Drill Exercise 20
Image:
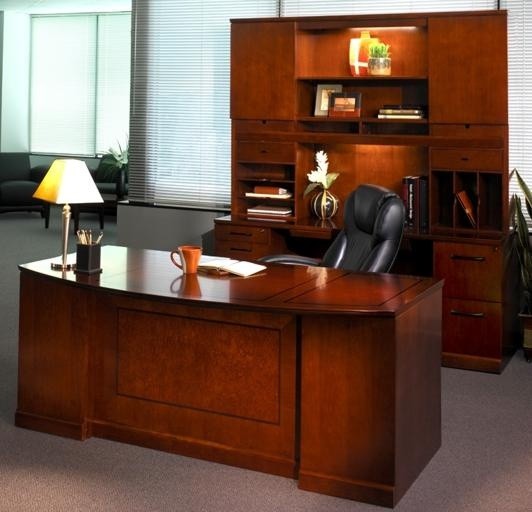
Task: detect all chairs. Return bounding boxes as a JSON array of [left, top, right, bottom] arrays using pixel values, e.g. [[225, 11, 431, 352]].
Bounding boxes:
[[254, 183, 407, 274], [87, 155, 126, 199], [0, 151, 51, 229]]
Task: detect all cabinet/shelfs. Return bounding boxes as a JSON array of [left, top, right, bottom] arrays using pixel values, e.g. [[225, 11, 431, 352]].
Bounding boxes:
[[230, 119, 312, 230], [229, 17, 298, 120], [432, 10, 508, 124], [428, 123, 510, 235], [215, 215, 274, 264], [298, 9, 431, 133], [436, 235, 512, 374]]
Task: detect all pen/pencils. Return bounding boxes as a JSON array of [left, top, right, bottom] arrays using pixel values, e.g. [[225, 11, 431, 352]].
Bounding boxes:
[[74, 228, 105, 245]]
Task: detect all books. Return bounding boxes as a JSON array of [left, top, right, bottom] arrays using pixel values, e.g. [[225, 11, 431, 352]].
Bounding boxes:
[[247, 205, 292, 215], [378, 104, 424, 120], [245, 192, 292, 199], [198, 255, 267, 277], [402, 175, 429, 234]]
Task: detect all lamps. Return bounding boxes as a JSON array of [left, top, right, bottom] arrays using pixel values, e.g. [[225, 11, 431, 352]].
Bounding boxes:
[[31, 155, 105, 270]]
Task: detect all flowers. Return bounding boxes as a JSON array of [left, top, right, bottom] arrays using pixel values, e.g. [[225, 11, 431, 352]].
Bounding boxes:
[[302, 149, 340, 194]]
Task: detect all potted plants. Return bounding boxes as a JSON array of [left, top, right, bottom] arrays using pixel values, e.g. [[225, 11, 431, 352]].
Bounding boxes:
[[509, 166, 532, 362], [367, 41, 391, 75]]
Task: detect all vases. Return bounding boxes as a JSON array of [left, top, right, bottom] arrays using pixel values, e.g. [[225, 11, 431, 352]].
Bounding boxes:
[[312, 189, 338, 220]]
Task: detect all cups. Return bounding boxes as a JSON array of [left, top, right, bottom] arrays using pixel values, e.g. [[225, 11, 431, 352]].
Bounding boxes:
[[171, 246, 202, 275]]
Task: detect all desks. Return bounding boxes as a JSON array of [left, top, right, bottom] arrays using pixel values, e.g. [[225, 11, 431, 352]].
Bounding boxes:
[[71, 193, 118, 235], [14, 243, 447, 510]]
